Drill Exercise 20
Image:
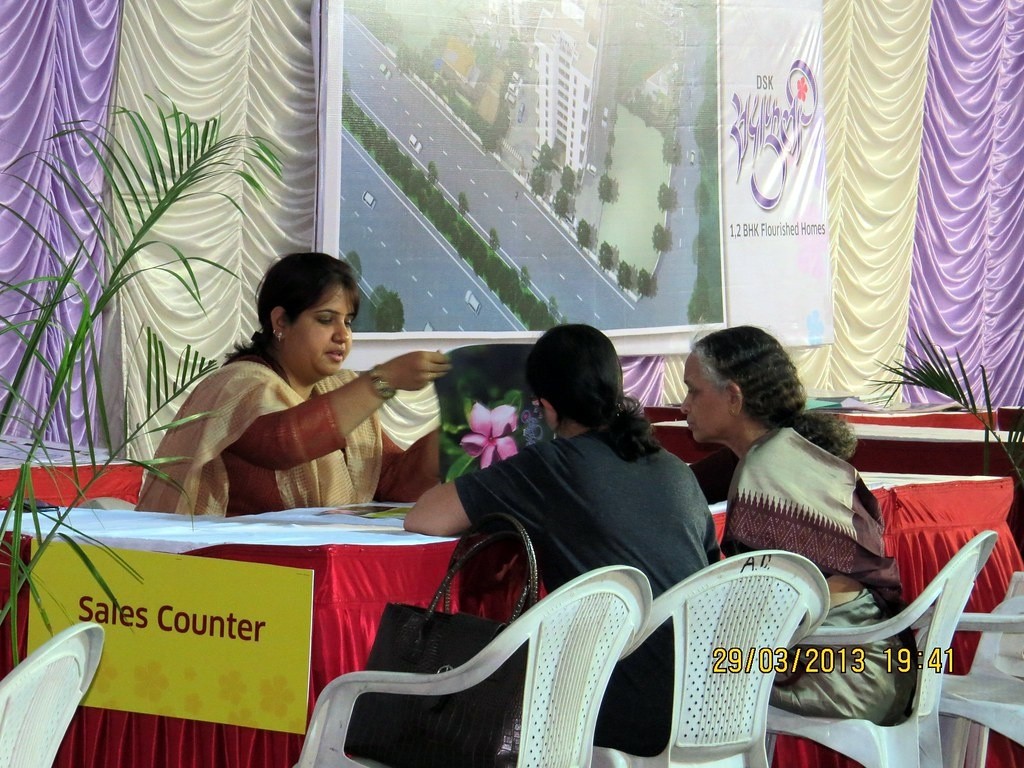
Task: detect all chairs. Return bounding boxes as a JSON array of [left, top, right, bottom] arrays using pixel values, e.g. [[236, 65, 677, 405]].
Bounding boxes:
[[0, 622, 105, 768], [293, 565, 654, 768], [916, 597, 1024, 768], [590, 549, 830, 768], [766, 530, 997, 768]]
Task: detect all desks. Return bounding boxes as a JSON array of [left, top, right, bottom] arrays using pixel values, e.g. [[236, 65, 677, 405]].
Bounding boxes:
[[0, 409, 1024, 764]]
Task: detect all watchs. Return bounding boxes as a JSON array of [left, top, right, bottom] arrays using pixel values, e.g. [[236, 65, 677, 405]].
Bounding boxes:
[[366, 370, 395, 401]]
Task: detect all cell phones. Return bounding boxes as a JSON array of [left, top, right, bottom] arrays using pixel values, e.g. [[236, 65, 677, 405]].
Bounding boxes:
[[23, 498, 59, 512]]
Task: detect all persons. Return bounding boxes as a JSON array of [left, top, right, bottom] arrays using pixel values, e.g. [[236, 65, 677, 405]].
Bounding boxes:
[[679, 325, 917, 725], [134, 252, 451, 537], [404, 324, 721, 752]]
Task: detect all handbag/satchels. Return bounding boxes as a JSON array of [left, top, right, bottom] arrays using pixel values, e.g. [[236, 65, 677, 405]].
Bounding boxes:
[[344, 512, 539, 768]]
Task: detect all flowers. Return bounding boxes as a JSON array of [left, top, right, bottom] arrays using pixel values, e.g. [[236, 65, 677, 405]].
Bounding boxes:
[[460, 402, 520, 470]]
